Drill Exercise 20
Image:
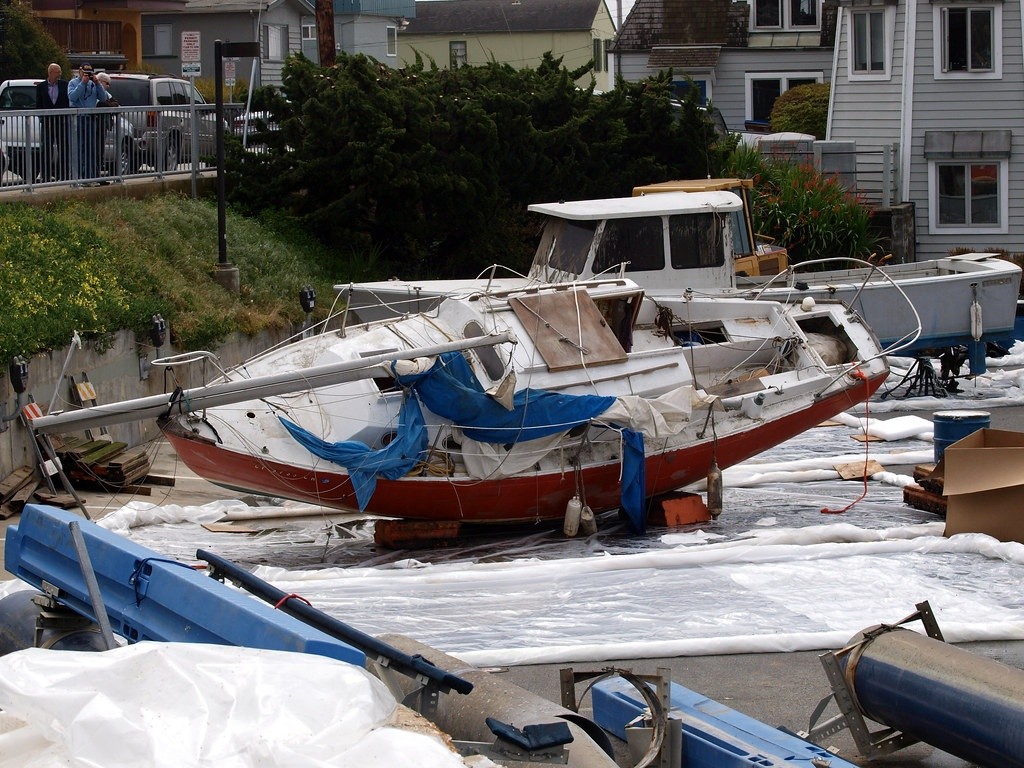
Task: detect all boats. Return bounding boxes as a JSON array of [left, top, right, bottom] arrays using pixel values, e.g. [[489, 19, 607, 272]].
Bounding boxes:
[[334, 191, 1023, 380], [158, 278, 890, 522]]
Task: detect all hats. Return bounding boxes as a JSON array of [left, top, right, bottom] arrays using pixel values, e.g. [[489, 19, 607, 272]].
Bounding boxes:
[[79, 63, 94, 73]]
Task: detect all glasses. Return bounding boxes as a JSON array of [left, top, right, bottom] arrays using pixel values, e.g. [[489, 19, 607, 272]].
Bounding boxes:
[[103, 80, 111, 86]]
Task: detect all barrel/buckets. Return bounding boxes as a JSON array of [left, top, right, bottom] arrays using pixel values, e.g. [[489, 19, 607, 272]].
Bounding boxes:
[[933, 410, 991, 464]]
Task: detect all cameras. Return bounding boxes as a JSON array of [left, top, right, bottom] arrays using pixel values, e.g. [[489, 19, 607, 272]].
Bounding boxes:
[[83, 69, 94, 77]]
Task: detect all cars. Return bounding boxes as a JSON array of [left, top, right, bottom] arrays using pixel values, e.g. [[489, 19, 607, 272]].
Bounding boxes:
[[232, 102, 281, 147]]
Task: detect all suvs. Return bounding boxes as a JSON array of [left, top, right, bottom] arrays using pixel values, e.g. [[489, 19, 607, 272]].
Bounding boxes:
[[95, 71, 230, 172]]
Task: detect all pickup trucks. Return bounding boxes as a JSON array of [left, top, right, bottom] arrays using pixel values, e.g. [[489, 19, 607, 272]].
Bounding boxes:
[[0, 79, 134, 178]]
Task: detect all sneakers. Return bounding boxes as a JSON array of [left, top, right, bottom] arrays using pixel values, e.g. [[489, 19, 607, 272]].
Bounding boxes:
[[80, 182, 100, 187]]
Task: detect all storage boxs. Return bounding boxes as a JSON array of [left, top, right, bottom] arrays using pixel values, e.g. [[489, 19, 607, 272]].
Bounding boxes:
[[944, 428, 1024, 544]]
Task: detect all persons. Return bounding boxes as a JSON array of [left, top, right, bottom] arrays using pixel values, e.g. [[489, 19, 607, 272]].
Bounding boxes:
[[36, 62, 120, 182]]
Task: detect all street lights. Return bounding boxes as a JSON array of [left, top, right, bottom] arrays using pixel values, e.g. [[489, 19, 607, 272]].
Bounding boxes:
[[214, 41, 262, 263]]
